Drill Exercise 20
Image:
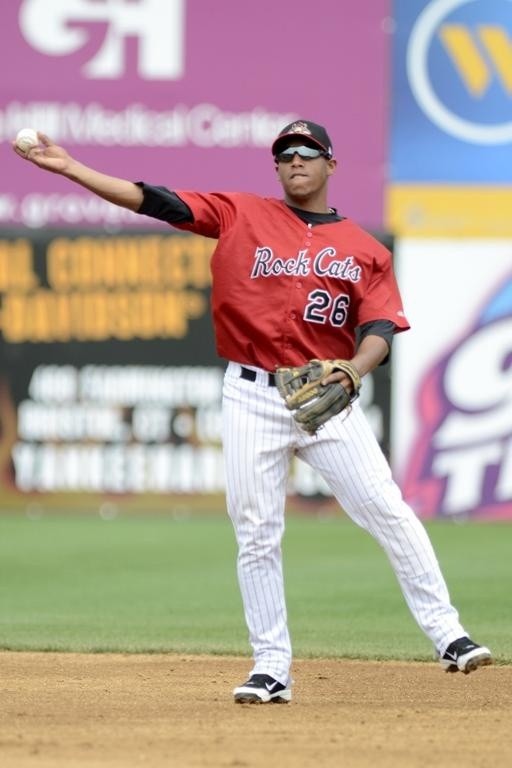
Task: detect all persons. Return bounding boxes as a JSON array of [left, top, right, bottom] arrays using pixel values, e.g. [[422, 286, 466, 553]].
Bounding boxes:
[[12, 120, 494, 705]]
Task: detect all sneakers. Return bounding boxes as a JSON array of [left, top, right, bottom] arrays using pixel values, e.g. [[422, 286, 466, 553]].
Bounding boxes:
[[440, 636, 493, 676], [234, 675, 293, 703]]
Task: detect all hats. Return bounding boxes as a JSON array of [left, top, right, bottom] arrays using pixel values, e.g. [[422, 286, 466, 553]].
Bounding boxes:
[[272, 120, 332, 157]]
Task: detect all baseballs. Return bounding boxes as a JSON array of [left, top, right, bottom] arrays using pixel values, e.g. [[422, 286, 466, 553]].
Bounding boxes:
[[16, 128, 40, 153]]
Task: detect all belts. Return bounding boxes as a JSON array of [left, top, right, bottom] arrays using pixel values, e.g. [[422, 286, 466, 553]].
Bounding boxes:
[[240, 366, 307, 388]]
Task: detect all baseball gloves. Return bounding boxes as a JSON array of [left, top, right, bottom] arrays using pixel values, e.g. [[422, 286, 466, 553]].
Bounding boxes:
[[274, 359, 361, 438]]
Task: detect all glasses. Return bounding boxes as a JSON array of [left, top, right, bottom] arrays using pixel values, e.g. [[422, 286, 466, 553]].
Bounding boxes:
[[274, 143, 330, 164]]
